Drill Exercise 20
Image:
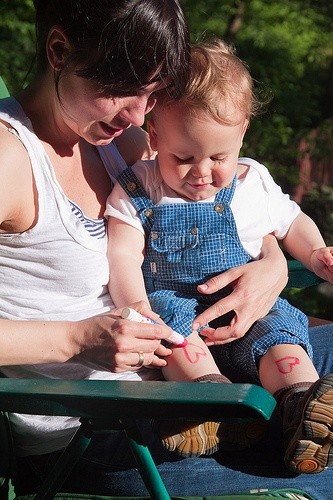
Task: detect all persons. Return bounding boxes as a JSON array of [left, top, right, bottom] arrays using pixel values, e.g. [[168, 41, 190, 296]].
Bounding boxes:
[[104, 37, 333, 473], [0, 0, 332, 500]]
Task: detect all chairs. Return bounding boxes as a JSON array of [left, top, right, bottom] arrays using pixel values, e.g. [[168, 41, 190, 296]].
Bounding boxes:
[[0, 260, 326, 500]]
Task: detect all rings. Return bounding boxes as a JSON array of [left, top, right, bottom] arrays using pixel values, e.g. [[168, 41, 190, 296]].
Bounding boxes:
[[135, 352, 145, 367]]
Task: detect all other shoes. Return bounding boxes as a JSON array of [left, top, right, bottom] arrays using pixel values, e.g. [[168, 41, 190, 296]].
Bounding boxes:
[[160, 373, 263, 458], [279, 372, 333, 475]]
